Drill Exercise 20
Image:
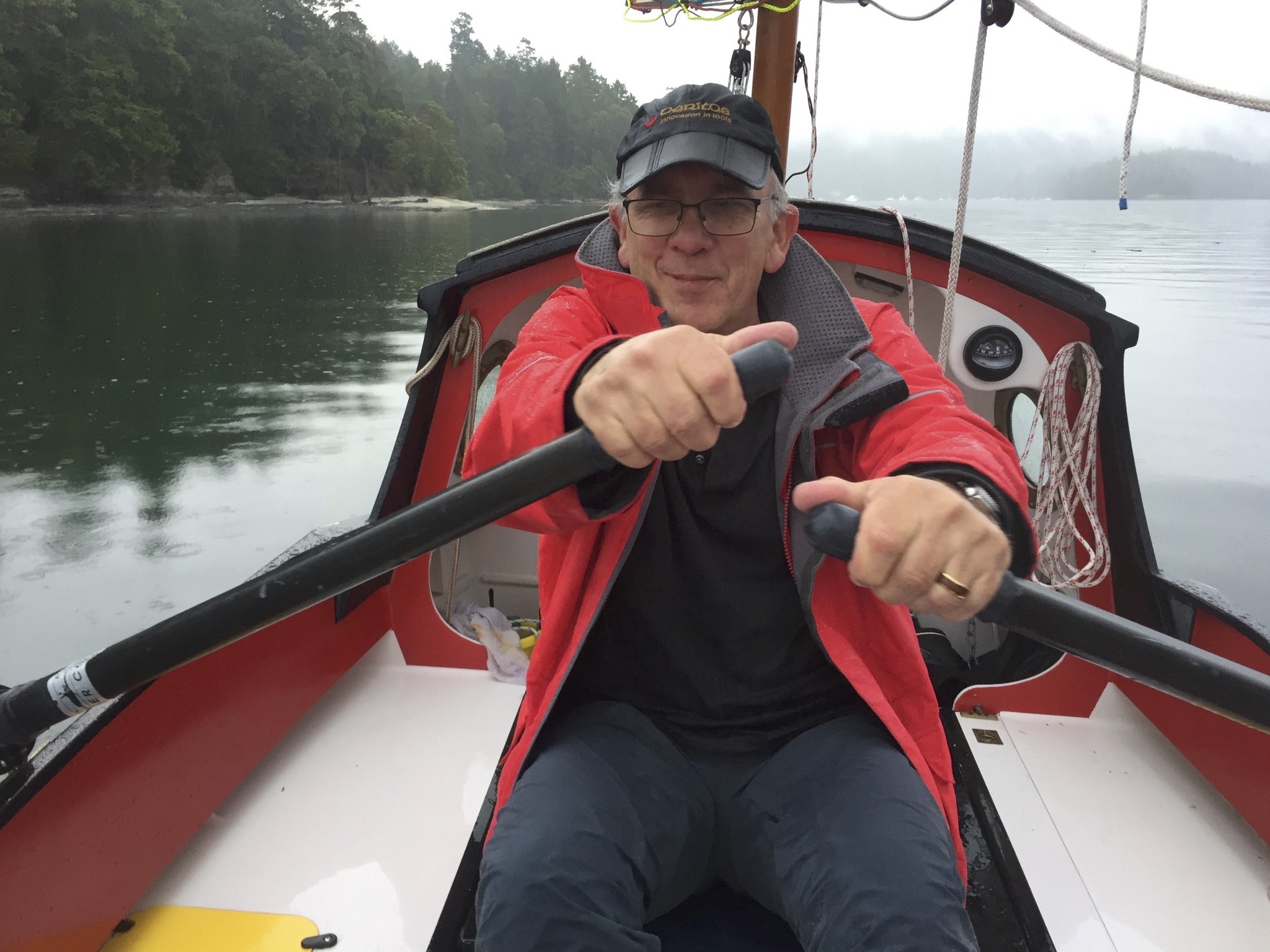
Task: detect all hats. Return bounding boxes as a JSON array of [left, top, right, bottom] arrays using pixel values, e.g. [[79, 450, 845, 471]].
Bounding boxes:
[[616, 83, 785, 191]]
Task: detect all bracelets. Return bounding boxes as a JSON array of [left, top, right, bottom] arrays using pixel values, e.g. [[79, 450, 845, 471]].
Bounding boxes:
[[931, 476, 1005, 535]]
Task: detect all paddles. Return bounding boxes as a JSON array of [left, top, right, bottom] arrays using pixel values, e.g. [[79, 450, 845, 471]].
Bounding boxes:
[[0, 339, 794, 753], [799, 500, 1270, 737]]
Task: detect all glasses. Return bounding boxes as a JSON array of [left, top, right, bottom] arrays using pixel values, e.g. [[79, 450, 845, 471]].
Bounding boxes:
[[620, 196, 777, 237]]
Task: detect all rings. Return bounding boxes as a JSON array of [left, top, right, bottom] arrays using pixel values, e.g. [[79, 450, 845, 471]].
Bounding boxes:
[[935, 571, 971, 599]]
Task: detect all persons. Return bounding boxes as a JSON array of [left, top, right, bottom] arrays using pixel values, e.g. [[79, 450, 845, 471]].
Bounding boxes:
[[460, 83, 1038, 952]]
